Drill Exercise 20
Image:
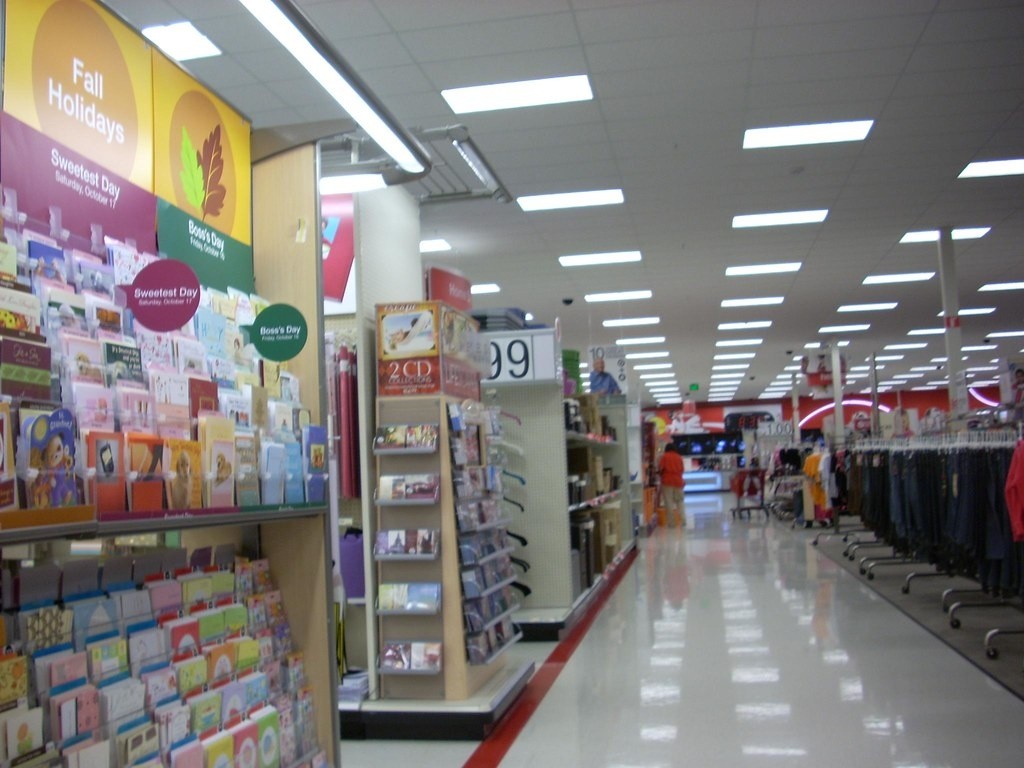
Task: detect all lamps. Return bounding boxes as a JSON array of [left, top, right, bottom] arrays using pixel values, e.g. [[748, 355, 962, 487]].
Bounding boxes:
[[235, 0, 430, 174]]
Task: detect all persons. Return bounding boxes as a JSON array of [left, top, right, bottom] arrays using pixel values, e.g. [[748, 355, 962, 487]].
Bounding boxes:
[[658, 443, 687, 530]]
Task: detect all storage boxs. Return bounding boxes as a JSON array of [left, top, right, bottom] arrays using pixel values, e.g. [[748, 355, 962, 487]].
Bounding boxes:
[[374, 403, 515, 671]]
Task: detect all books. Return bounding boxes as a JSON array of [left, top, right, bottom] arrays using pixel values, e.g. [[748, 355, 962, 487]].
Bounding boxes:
[[371, 423, 440, 671], [0, 228, 327, 768]]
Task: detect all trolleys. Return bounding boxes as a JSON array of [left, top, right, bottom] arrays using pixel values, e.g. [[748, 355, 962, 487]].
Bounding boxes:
[[730, 469, 769, 520]]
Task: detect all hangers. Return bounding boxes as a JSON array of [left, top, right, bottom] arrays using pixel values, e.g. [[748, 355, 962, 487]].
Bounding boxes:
[[854, 430, 1024, 455], [486, 389, 533, 598]]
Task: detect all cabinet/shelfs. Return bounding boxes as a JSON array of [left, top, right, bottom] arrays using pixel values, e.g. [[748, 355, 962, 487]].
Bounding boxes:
[[375, 393, 524, 703], [0, 139, 341, 767], [564, 430, 623, 605]]
